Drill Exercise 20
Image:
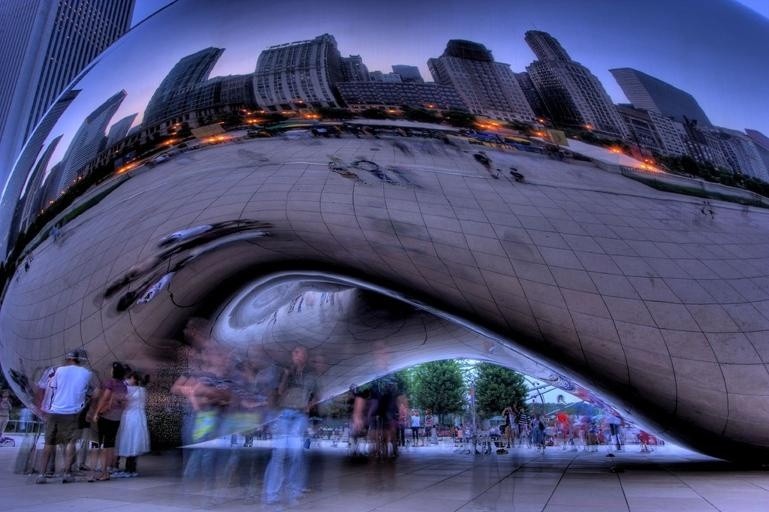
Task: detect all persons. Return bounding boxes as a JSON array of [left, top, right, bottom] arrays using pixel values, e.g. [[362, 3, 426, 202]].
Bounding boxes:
[[1, 314, 663, 512], [312, 120, 524, 192]]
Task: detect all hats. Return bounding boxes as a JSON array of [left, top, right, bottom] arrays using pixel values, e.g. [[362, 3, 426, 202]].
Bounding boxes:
[[67, 351, 87, 360]]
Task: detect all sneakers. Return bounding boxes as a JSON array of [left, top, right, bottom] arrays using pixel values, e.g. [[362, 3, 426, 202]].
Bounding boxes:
[[35, 464, 139, 484]]
